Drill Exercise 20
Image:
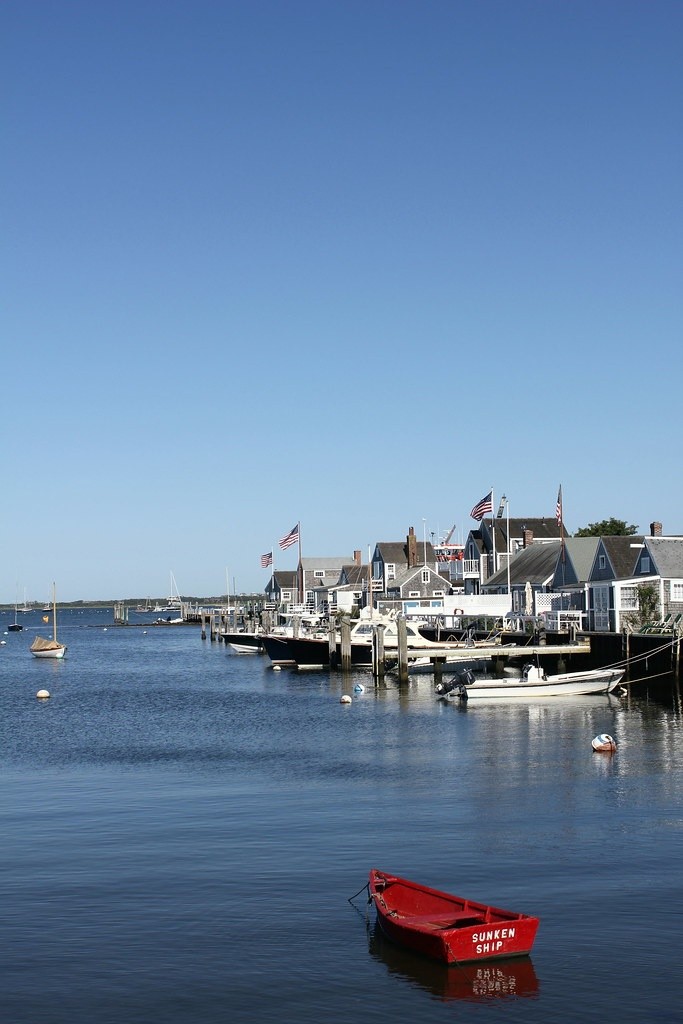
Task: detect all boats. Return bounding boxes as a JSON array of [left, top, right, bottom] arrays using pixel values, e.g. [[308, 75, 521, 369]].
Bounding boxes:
[[436, 656, 627, 705], [218, 629, 267, 656], [381, 645, 499, 674], [367, 865, 541, 967], [260, 615, 502, 663]]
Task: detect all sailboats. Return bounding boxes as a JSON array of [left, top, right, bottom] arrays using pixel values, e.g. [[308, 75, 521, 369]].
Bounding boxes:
[[84, 566, 253, 625], [30, 582, 66, 657], [18, 586, 32, 613], [41, 590, 52, 611], [8, 589, 23, 632]]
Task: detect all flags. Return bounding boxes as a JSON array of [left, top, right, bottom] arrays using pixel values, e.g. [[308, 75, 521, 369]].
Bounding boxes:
[[278, 523, 299, 551], [470, 491, 492, 521], [261, 552, 272, 568], [555, 489, 562, 526]]
